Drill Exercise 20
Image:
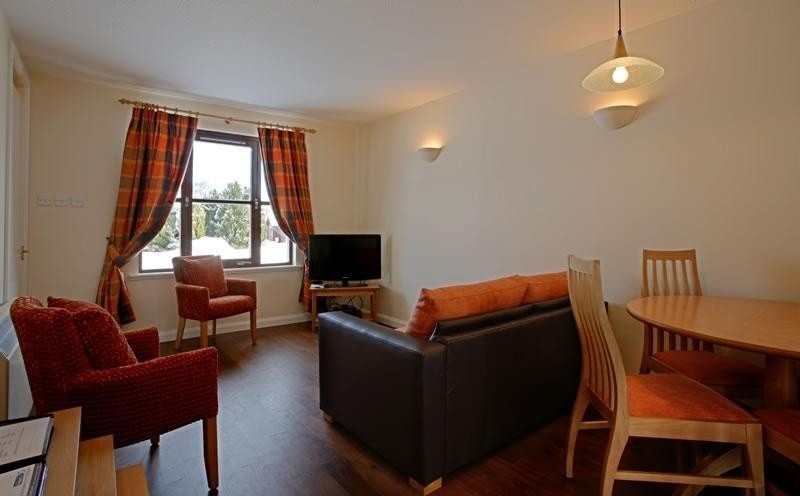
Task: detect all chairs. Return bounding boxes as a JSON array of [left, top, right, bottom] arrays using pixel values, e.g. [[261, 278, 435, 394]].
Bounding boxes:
[[9, 293, 222, 496], [171, 253, 257, 351], [565, 249, 800, 495]]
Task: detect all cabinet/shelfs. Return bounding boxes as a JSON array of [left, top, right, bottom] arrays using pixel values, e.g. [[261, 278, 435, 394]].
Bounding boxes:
[[0, 406, 152, 496]]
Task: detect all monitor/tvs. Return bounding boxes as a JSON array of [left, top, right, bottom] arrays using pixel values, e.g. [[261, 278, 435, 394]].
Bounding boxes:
[[307, 233, 383, 287]]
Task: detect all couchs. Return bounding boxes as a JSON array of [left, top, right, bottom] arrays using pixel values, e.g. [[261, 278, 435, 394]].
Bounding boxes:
[[315, 272, 609, 493]]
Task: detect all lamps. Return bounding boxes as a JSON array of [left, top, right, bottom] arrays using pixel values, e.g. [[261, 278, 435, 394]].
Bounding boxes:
[[417, 147, 441, 163], [580, 0, 666, 131]]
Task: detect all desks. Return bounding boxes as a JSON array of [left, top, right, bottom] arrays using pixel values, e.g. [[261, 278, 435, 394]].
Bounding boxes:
[[309, 284, 380, 333]]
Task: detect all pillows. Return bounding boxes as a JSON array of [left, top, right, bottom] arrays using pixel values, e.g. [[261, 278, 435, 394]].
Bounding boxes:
[[181, 255, 228, 298], [46, 297, 137, 370]]
